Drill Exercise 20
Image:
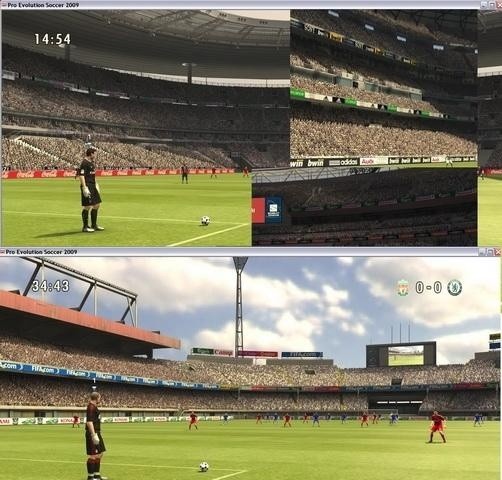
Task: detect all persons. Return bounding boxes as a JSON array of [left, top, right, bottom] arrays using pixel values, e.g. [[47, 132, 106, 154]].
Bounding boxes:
[[424, 409, 445, 443], [3, 41, 251, 179], [179, 161, 190, 185], [85, 391, 110, 480], [77, 147, 105, 234], [254, 9, 502, 247], [0, 315, 500, 429], [188, 411, 199, 431]]
